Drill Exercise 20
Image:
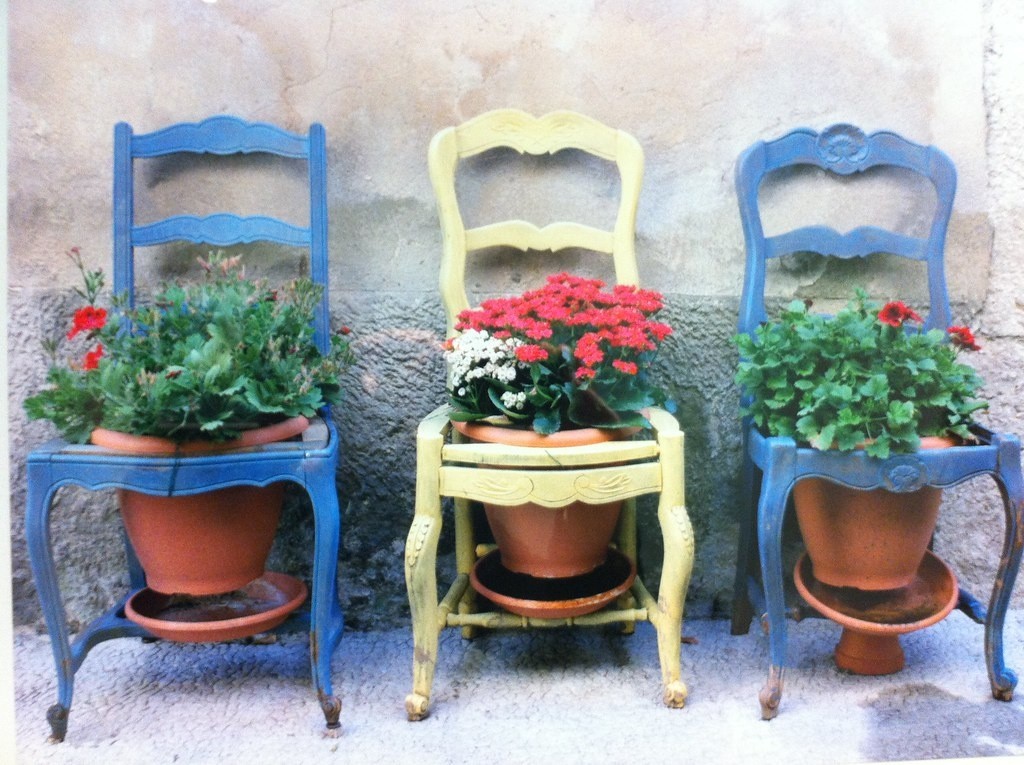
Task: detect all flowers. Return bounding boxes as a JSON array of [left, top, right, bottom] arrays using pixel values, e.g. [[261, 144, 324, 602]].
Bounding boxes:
[[731, 282, 991, 450], [20, 241, 362, 449], [437, 273, 674, 441]]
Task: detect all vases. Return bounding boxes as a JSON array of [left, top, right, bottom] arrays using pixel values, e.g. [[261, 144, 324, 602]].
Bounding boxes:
[[83, 411, 308, 598], [446, 415, 652, 579], [787, 434, 959, 590]]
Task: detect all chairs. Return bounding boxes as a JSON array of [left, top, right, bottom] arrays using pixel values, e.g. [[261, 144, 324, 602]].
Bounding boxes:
[[24, 118, 348, 738], [734, 122, 1024, 720], [405, 103, 696, 720]]
